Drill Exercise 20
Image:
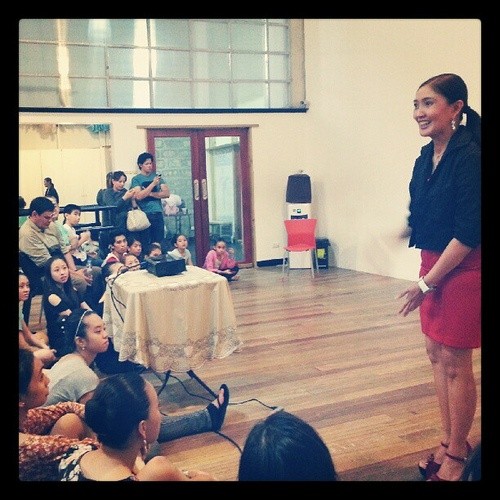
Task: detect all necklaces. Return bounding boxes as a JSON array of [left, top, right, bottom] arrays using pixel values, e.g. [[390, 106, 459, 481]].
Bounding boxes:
[[433, 147, 446, 160]]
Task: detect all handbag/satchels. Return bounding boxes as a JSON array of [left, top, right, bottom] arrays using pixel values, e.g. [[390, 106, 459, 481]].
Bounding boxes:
[[126, 206, 151, 233]]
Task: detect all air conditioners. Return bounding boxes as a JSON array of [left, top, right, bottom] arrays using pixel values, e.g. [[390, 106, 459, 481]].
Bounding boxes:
[[286, 174, 313, 269]]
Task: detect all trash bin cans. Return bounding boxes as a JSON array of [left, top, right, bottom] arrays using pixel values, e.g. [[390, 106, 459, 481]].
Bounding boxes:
[[313, 237, 331, 268]]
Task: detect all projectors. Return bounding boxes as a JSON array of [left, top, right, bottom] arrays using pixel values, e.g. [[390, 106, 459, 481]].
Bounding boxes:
[[144, 252, 187, 277]]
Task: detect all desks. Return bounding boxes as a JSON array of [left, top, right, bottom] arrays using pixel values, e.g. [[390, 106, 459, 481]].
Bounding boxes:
[[107, 264, 232, 406]]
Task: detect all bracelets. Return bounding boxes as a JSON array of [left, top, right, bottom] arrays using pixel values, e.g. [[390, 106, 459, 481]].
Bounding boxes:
[[418, 275, 436, 294]]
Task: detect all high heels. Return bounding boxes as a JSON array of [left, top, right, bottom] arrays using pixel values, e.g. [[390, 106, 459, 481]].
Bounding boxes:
[[429, 440, 472, 481], [419, 441, 448, 477]]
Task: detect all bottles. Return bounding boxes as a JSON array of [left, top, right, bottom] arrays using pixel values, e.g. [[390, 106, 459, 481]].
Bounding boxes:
[[86, 259, 92, 286]]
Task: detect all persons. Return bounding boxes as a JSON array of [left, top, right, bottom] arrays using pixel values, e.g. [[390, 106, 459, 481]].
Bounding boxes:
[[41, 255, 148, 376], [102, 170, 141, 246], [128, 152, 169, 256], [40, 308, 229, 464], [43, 177, 59, 205], [18, 272, 58, 370], [18, 349, 104, 480], [203, 238, 239, 283], [237, 410, 337, 481], [41, 194, 104, 315], [61, 217, 195, 275], [59, 204, 106, 267], [387, 74, 482, 482], [19, 196, 25, 210], [97, 172, 113, 257], [19, 197, 86, 304], [57, 374, 219, 481]]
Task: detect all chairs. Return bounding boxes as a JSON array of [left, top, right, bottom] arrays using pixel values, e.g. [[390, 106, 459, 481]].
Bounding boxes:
[[279, 219, 320, 279], [19, 251, 47, 330]]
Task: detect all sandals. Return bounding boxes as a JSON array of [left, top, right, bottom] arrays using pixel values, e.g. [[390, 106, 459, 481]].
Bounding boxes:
[[206, 384, 229, 431]]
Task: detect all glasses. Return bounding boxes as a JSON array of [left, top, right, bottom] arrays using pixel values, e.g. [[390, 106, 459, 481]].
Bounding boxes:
[[43, 212, 57, 218]]
